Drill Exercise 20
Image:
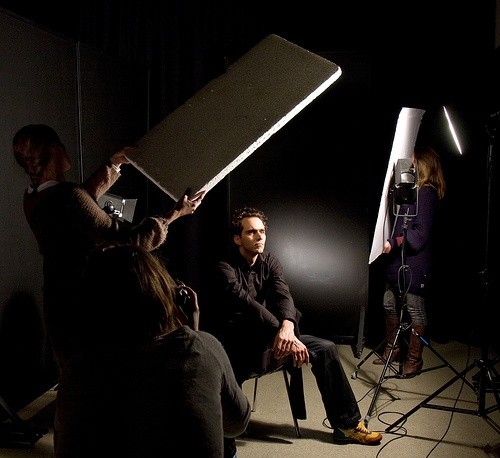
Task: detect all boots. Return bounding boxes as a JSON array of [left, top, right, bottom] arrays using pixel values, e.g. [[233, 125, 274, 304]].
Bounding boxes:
[[373, 315, 402, 364], [396, 323, 427, 379]]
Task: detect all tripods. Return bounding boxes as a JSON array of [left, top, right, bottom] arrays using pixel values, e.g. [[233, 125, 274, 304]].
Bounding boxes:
[[352, 216, 500, 432]]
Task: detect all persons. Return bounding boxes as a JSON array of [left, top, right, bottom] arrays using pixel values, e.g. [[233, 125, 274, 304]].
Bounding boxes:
[[206, 206, 383, 445], [372, 146, 445, 378], [11, 123, 205, 401], [53, 245, 251, 458]]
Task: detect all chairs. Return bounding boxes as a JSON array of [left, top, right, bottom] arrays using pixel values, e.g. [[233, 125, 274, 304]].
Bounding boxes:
[[250, 365, 301, 439]]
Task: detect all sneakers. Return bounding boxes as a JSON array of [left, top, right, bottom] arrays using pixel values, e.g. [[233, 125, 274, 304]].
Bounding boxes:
[[333, 418, 383, 445]]
